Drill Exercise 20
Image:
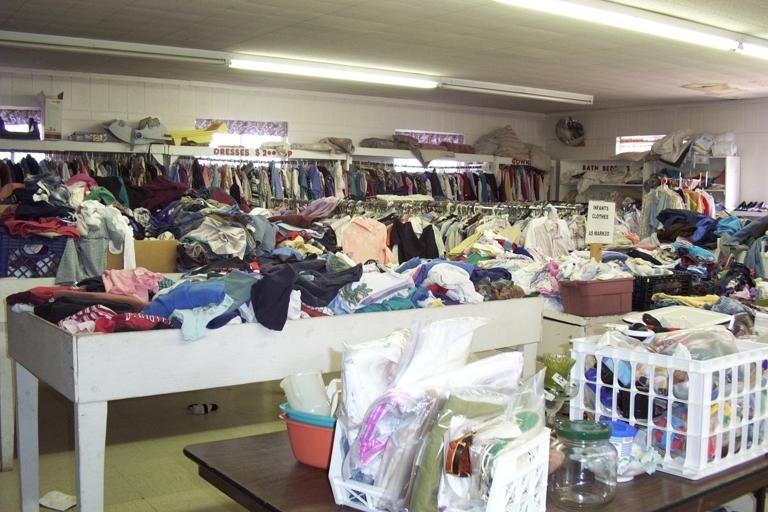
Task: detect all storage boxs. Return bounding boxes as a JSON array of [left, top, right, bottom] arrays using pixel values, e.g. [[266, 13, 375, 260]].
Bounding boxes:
[[557, 272, 694, 318]]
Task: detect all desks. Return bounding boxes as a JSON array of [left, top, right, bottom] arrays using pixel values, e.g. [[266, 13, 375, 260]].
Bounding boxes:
[[183, 402, 766, 512], [6, 293, 545, 511]]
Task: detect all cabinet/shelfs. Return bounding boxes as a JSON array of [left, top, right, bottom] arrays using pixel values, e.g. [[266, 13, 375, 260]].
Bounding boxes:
[[558, 156, 741, 239]]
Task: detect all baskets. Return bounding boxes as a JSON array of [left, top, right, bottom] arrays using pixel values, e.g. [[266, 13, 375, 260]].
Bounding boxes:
[[632, 271, 691, 311], [0, 235, 67, 279], [569, 334, 768, 481]]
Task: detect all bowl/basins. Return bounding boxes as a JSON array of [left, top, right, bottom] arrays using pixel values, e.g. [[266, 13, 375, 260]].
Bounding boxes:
[[277, 404, 337, 468]]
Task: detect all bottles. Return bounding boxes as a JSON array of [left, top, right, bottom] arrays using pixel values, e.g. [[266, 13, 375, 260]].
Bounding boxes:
[[550, 419, 618, 511]]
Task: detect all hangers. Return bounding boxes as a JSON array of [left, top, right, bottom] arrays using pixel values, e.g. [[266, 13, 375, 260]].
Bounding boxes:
[[38, 152, 344, 178], [325, 200, 569, 237]]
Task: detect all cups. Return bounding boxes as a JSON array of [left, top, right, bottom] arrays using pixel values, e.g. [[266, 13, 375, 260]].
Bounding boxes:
[[278, 368, 331, 419], [542, 352, 576, 390]]
